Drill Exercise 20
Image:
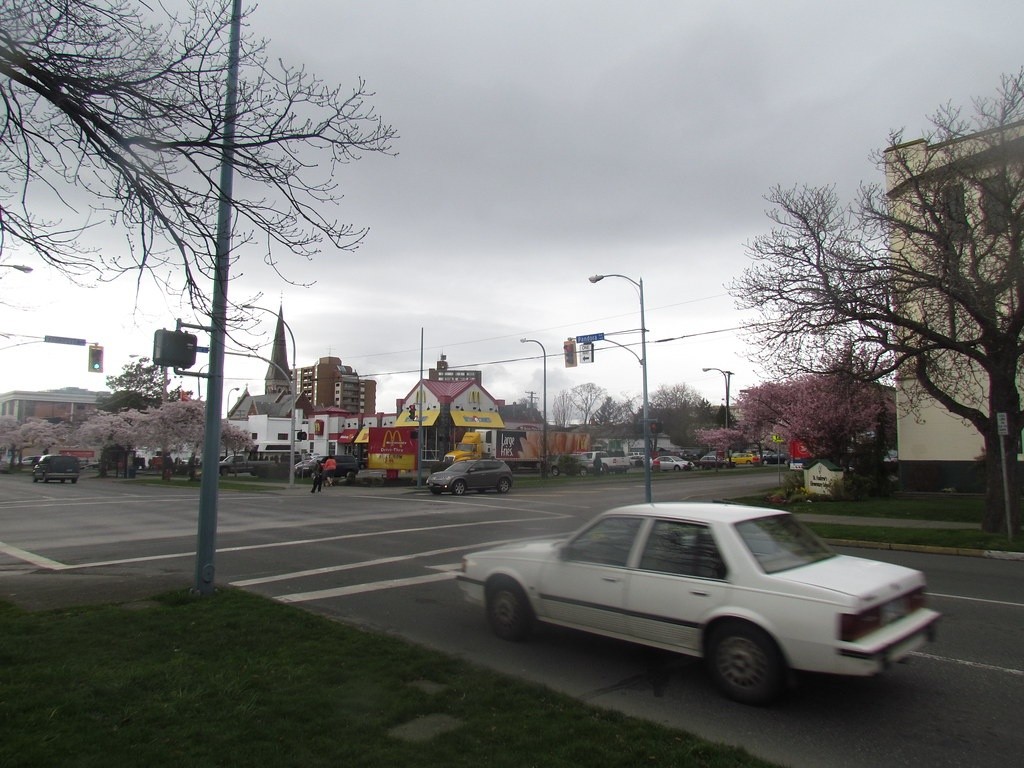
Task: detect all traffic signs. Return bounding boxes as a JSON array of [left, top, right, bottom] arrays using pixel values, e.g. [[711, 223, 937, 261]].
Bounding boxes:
[[580, 343, 593, 364]]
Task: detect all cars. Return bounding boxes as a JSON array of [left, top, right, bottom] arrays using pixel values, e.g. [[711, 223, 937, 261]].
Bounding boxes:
[[653, 456, 695, 473], [726, 453, 755, 465], [753, 450, 786, 465], [457, 499, 942, 716], [628, 451, 652, 468], [31, 453, 80, 484], [22, 455, 41, 467], [551, 453, 603, 478], [693, 455, 729, 469]]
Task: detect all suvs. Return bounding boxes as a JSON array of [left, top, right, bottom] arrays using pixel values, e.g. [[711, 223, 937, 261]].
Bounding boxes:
[[424, 456, 514, 495]]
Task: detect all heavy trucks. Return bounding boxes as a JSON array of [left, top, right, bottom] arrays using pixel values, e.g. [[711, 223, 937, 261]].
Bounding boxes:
[[442, 425, 592, 476]]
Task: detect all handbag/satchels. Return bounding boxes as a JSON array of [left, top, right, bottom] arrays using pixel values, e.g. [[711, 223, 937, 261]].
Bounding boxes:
[[311, 473, 316, 480]]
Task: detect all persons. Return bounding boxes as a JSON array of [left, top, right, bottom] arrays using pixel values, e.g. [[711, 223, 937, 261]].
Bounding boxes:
[[310, 454, 337, 494]]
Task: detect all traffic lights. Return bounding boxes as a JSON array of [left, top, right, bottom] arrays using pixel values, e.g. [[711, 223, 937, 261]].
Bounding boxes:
[[563, 341, 577, 369], [408, 405, 415, 420], [88, 345, 103, 374]]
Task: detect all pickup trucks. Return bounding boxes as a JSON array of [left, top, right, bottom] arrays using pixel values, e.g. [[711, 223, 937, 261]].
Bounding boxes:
[[242, 303, 298, 488], [576, 451, 631, 476], [219, 454, 276, 477]]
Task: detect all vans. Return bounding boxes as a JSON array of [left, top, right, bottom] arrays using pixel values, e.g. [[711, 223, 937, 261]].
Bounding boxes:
[[296, 454, 359, 480]]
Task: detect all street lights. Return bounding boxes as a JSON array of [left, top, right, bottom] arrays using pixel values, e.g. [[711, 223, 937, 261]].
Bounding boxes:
[[589, 274, 652, 503], [520, 338, 546, 480], [703, 367, 728, 430]]
